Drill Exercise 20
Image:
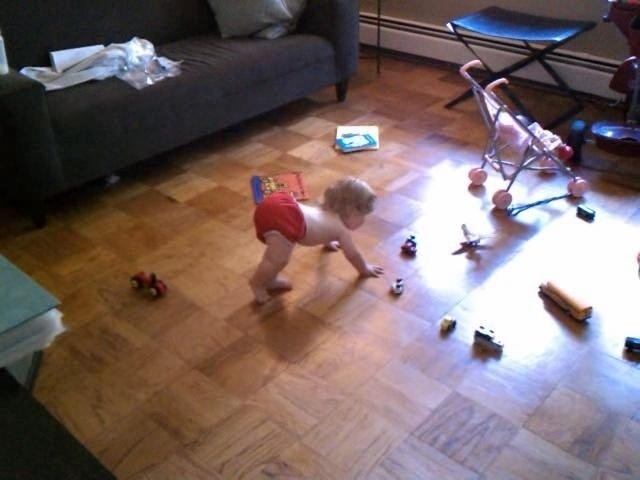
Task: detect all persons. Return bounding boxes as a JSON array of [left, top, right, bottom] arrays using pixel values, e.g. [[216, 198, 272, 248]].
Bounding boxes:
[[247, 174, 385, 305]]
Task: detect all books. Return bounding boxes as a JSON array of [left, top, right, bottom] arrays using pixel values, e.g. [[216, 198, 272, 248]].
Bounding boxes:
[[335, 125, 380, 151], [335, 133, 377, 153], [250, 170, 310, 205]]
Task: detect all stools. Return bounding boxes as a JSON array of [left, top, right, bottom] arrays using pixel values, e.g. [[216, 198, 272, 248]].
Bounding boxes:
[[444, 7, 598, 134]]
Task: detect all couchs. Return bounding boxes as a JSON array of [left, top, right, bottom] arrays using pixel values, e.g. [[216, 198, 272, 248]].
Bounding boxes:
[[0, 1, 360, 229]]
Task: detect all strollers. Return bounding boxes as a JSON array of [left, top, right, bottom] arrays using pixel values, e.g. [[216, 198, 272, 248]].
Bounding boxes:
[[459, 58, 588, 211]]
